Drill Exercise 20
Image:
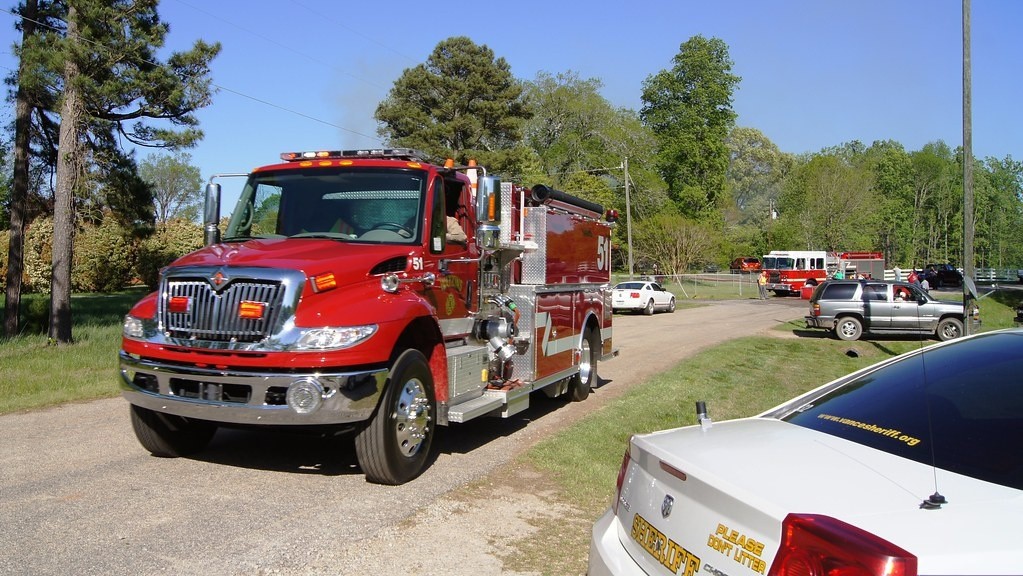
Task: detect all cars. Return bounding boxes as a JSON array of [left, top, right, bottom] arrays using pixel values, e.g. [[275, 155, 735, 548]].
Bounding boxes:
[[609, 280, 677, 316], [906, 263, 963, 286], [729, 257, 760, 273], [588, 323, 1023, 576], [704, 264, 718, 272]]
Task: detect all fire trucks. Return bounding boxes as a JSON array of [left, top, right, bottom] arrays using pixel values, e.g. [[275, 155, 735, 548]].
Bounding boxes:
[[115, 146, 620, 487], [760, 249, 887, 298]]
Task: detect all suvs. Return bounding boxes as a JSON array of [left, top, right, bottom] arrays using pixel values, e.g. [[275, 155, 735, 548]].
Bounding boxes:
[[803, 277, 981, 344]]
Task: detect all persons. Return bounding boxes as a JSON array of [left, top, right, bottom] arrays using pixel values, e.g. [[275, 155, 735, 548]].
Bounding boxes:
[[829, 268, 876, 280], [893, 266, 902, 282], [895, 289, 909, 302], [397, 215, 468, 243], [907, 264, 937, 293], [757, 271, 770, 300]]
